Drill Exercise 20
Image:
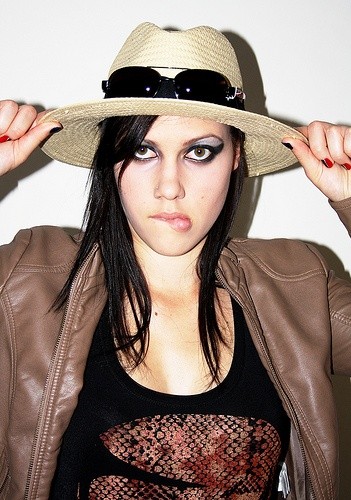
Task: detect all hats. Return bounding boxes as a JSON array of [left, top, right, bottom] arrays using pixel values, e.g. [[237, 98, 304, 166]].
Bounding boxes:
[[38, 22, 314, 176]]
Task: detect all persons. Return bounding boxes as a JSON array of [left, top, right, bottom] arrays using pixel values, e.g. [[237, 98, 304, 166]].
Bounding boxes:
[[0, 22, 351, 500]]
